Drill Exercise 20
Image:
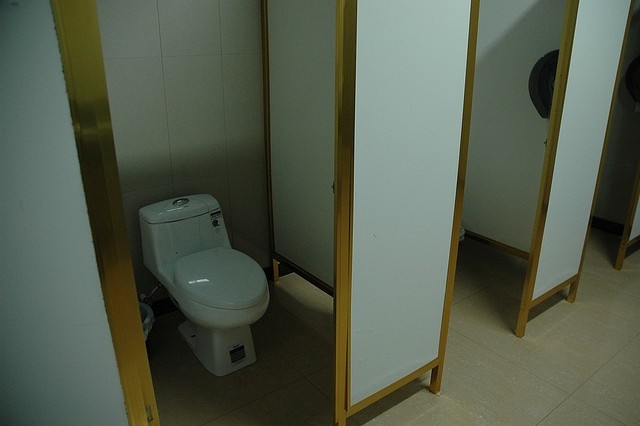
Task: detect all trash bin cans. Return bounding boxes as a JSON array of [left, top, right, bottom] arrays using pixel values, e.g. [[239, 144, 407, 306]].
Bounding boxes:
[[138, 301, 155, 339]]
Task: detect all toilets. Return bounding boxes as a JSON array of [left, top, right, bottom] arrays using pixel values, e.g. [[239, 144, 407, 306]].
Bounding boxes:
[[139, 194, 271, 377]]
